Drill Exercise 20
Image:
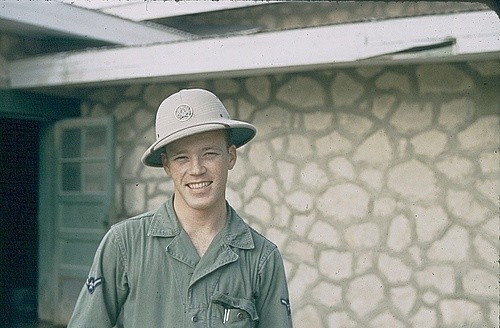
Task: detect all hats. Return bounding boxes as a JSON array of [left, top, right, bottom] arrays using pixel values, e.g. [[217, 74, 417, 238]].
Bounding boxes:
[[141, 88, 256, 167]]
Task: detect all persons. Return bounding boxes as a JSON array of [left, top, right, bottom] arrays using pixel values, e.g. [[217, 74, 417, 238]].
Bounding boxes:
[[67, 89, 294, 328]]
